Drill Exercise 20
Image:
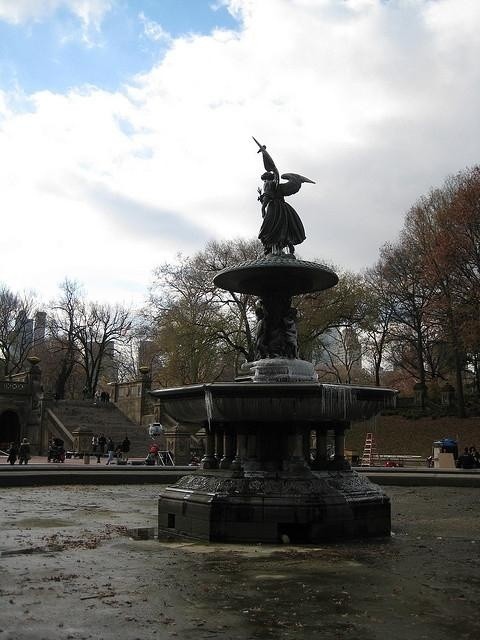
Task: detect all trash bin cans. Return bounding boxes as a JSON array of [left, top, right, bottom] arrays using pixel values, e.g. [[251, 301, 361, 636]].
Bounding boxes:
[[83, 456, 89, 465]]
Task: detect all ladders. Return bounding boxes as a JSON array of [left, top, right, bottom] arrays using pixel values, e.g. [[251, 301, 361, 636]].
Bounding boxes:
[[361, 433, 372, 467]]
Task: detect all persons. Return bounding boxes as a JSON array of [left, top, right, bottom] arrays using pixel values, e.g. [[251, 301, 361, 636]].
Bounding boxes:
[[101, 390, 106, 405], [17, 438, 31, 464], [253, 307, 266, 360], [252, 134, 315, 261], [95, 390, 101, 403], [282, 308, 301, 359], [149, 444, 158, 459], [91, 433, 130, 464], [469, 446, 480, 469], [106, 392, 110, 404], [457, 446, 470, 468], [7, 442, 17, 464]]
